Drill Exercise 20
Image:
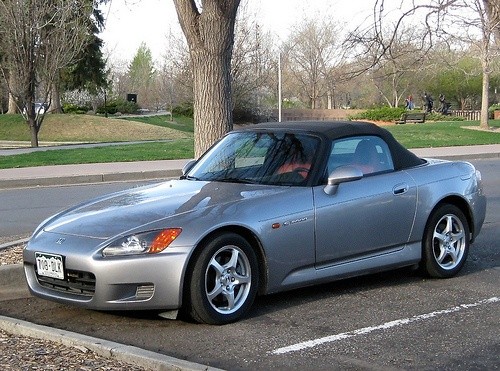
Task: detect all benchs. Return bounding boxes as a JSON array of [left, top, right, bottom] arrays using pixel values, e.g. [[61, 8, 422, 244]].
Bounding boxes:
[[395, 113, 425, 125]]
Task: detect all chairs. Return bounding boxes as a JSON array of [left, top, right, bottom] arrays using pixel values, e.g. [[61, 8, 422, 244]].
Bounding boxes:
[[339, 140, 386, 175], [275, 142, 316, 184]]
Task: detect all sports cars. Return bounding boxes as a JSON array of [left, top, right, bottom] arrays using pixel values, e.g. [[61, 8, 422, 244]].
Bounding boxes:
[[21, 119, 489, 326]]
